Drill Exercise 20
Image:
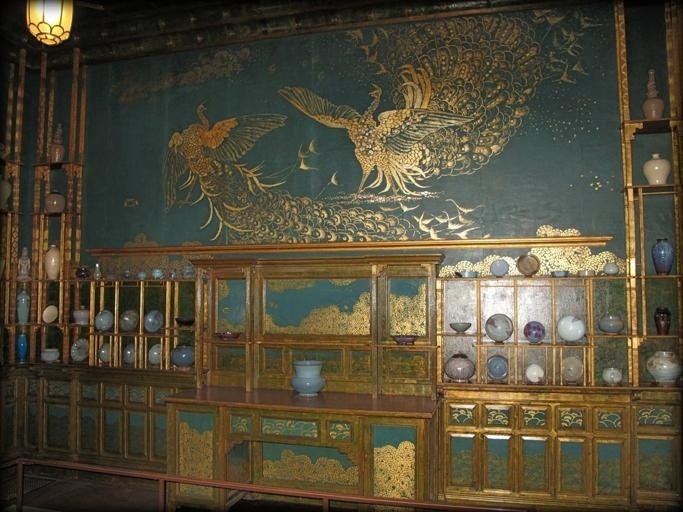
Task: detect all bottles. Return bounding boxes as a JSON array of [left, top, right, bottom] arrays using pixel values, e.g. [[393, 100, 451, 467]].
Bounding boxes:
[[44, 244, 60, 280]]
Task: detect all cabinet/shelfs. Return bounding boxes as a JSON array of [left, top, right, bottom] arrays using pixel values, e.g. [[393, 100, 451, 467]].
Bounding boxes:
[[0, 156, 195, 483], [161, 252, 446, 510], [437, 116, 680, 511]]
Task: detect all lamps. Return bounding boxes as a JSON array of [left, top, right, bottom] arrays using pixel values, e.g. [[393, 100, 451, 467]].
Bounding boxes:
[[22, 1, 75, 49]]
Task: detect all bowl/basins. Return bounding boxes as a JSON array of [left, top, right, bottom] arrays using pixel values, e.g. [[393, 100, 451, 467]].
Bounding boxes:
[[450, 323, 471, 332]]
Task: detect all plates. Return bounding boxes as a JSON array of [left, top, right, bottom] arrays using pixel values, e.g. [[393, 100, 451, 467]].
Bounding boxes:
[[485, 312, 586, 383], [215, 333, 242, 339], [390, 335, 419, 343], [95, 310, 164, 368], [490, 255, 540, 276], [42, 305, 58, 324], [70, 337, 88, 362]]
